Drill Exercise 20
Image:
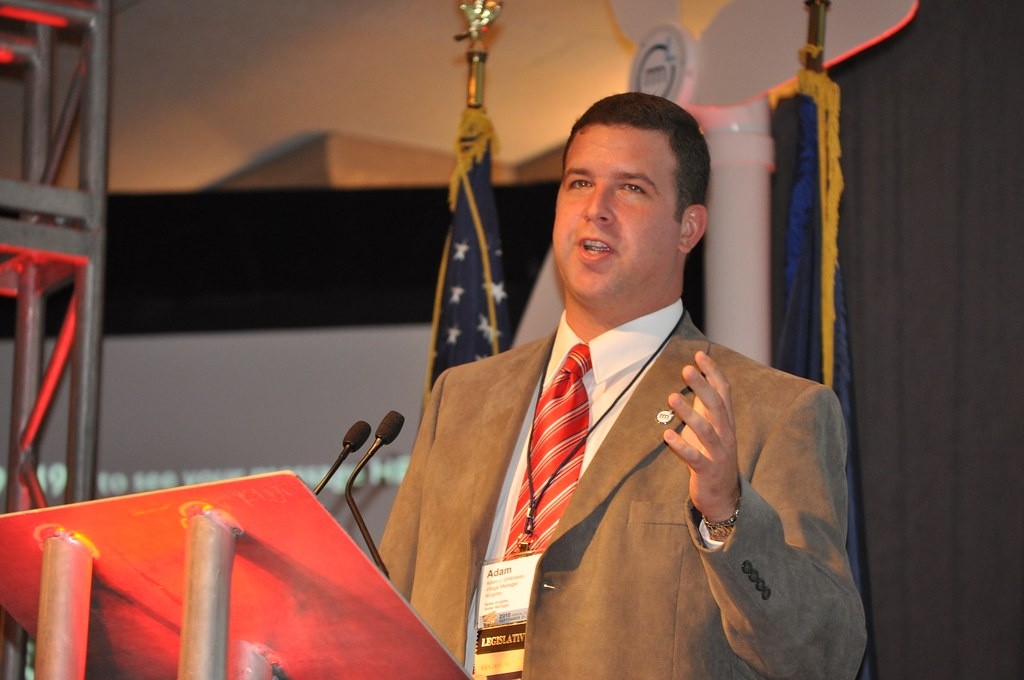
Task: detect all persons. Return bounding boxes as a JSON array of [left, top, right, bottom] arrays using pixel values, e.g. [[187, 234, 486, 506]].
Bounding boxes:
[[376, 92, 867, 680]]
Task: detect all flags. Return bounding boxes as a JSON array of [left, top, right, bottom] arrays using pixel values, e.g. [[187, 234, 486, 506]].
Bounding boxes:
[[421, 0, 509, 420], [768, 1, 879, 680]]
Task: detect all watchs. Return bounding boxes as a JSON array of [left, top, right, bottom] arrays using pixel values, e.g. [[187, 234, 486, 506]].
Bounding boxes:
[[702, 496, 742, 533]]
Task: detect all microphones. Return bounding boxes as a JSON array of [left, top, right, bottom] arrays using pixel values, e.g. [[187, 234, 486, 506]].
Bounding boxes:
[[345, 411, 405, 581], [313, 421, 371, 496]]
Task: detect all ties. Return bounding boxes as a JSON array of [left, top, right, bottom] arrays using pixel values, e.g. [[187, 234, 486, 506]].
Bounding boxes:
[[500, 342, 591, 559]]
